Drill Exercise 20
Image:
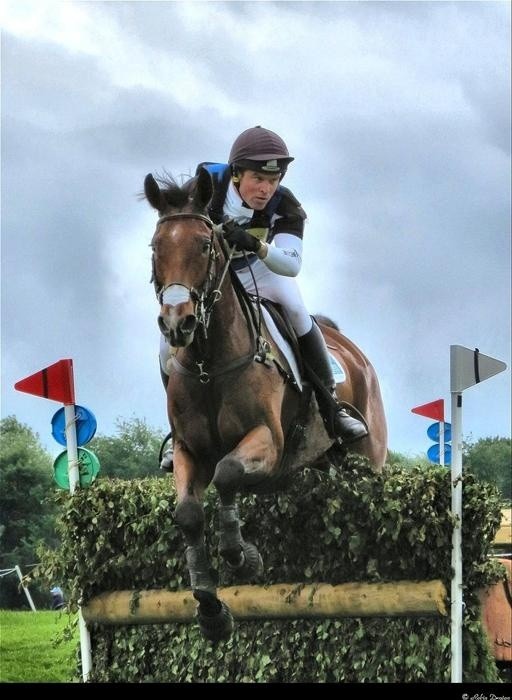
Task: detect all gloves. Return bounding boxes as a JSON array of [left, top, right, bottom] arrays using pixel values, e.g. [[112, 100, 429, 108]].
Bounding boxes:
[[228, 228, 260, 253], [221, 219, 241, 239]]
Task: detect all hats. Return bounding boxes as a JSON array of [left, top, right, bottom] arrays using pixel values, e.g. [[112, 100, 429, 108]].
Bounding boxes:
[[228, 125, 295, 162]]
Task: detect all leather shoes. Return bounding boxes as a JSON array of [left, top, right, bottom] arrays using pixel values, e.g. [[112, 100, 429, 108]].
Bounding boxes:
[[160, 442, 177, 473]]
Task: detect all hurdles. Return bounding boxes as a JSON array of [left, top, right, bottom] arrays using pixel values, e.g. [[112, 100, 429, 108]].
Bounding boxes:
[[16, 346, 507, 683]]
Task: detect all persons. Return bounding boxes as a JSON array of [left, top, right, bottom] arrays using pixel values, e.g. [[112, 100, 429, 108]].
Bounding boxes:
[[159, 126, 367, 472]]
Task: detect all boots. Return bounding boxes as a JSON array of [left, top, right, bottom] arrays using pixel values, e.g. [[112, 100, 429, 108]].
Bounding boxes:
[[298, 314, 368, 445]]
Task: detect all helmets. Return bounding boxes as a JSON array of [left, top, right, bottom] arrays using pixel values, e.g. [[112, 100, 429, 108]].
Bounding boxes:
[[231, 158, 288, 183]]
